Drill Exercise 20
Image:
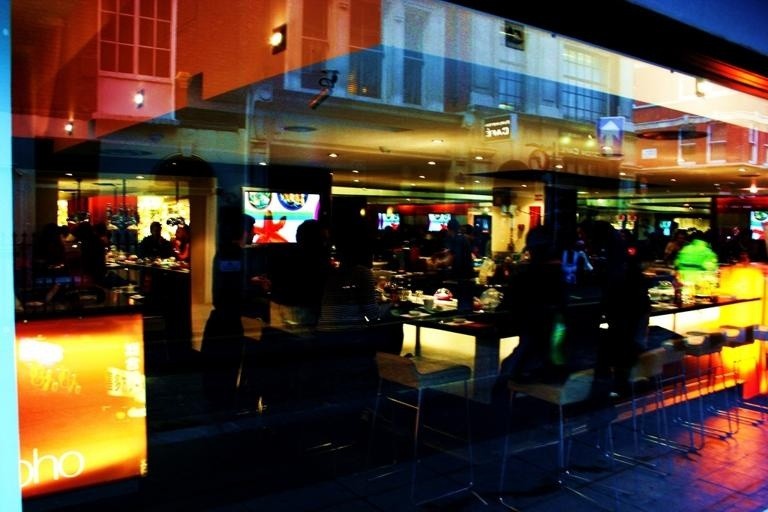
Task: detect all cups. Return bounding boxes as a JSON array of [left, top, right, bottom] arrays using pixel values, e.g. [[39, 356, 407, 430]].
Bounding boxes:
[[422, 299, 434, 311]]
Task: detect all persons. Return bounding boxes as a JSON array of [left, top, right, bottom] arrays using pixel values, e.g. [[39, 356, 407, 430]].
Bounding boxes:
[[10, 208, 768, 483]]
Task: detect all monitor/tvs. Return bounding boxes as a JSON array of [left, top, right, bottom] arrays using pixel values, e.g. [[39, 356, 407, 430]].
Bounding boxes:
[[749, 209, 768, 244], [375, 210, 401, 234], [425, 210, 451, 236], [237, 184, 323, 248]]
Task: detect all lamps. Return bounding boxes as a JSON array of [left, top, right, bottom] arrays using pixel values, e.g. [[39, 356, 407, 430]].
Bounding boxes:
[[306, 69, 340, 111]]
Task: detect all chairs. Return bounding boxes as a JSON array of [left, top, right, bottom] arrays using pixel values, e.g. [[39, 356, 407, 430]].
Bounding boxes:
[[233, 314, 282, 414]]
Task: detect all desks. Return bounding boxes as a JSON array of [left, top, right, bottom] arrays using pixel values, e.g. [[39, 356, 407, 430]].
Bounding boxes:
[[116, 258, 190, 306]]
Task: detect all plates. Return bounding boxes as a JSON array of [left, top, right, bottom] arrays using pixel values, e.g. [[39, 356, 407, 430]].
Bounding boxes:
[[453, 319, 466, 323], [399, 312, 431, 319]]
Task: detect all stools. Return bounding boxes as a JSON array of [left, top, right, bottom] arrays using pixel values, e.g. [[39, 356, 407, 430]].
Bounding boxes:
[[497, 323, 768, 512], [367, 351, 474, 512]]
